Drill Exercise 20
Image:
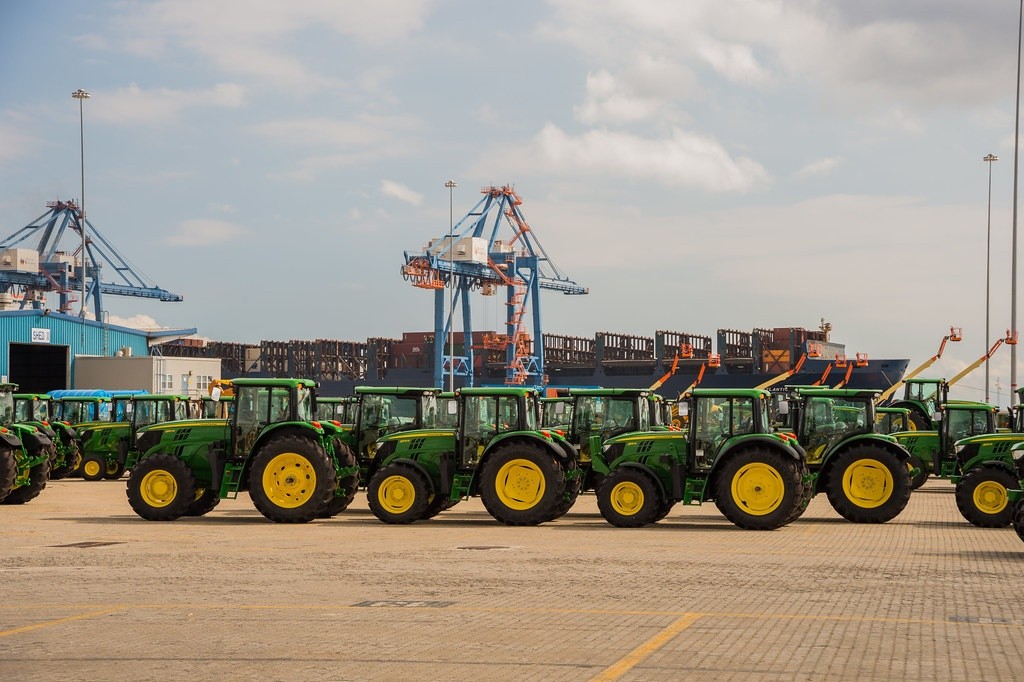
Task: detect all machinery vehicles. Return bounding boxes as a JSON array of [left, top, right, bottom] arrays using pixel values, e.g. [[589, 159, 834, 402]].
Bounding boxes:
[[0, 377, 1024, 542]]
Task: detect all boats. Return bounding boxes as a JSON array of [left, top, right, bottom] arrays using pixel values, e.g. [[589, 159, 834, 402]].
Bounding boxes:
[[0, 186, 916, 404]]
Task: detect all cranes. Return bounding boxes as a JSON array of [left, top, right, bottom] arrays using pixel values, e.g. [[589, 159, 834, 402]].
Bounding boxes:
[[400, 184, 591, 392], [0, 200, 187, 319]]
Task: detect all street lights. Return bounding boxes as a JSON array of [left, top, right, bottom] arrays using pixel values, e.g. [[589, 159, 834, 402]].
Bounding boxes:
[[72, 88, 91, 354], [981, 153, 999, 403], [444, 180, 459, 391]]
